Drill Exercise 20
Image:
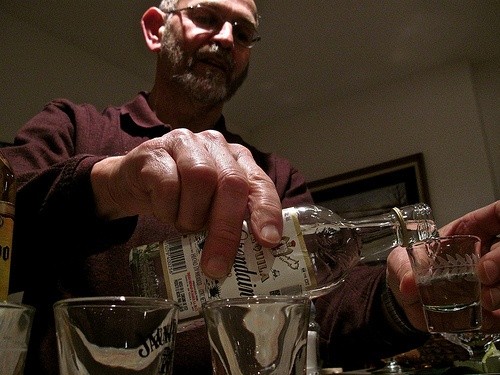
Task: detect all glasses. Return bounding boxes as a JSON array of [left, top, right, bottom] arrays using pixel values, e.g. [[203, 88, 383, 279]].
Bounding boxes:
[[164, 5, 262, 49]]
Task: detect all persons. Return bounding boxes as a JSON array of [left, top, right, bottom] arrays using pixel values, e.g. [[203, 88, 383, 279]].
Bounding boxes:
[[0, 0, 500, 375]]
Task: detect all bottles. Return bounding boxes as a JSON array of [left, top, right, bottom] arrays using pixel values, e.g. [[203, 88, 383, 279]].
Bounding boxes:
[[129, 203, 437, 334], [306, 321, 320, 375]]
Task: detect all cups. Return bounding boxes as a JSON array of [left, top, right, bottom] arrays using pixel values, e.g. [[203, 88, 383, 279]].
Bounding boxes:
[[52, 298, 179, 375], [201, 294, 310, 375], [406, 236, 481, 334], [0, 303, 35, 375]]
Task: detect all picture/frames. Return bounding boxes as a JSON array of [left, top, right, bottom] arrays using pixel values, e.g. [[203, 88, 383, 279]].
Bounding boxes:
[[304, 153, 431, 221]]
